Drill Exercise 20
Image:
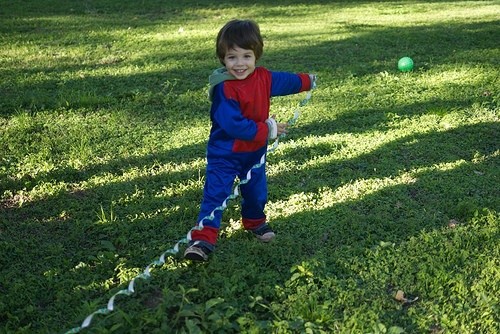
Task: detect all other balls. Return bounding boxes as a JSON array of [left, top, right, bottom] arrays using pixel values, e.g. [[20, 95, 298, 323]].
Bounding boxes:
[[397, 55, 415, 73]]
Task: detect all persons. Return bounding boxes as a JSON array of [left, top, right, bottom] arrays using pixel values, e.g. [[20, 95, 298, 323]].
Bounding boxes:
[[184, 20, 316, 260]]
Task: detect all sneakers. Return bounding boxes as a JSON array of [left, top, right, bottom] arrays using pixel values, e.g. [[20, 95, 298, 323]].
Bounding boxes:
[[182, 243, 210, 260], [251, 223, 275, 242]]
